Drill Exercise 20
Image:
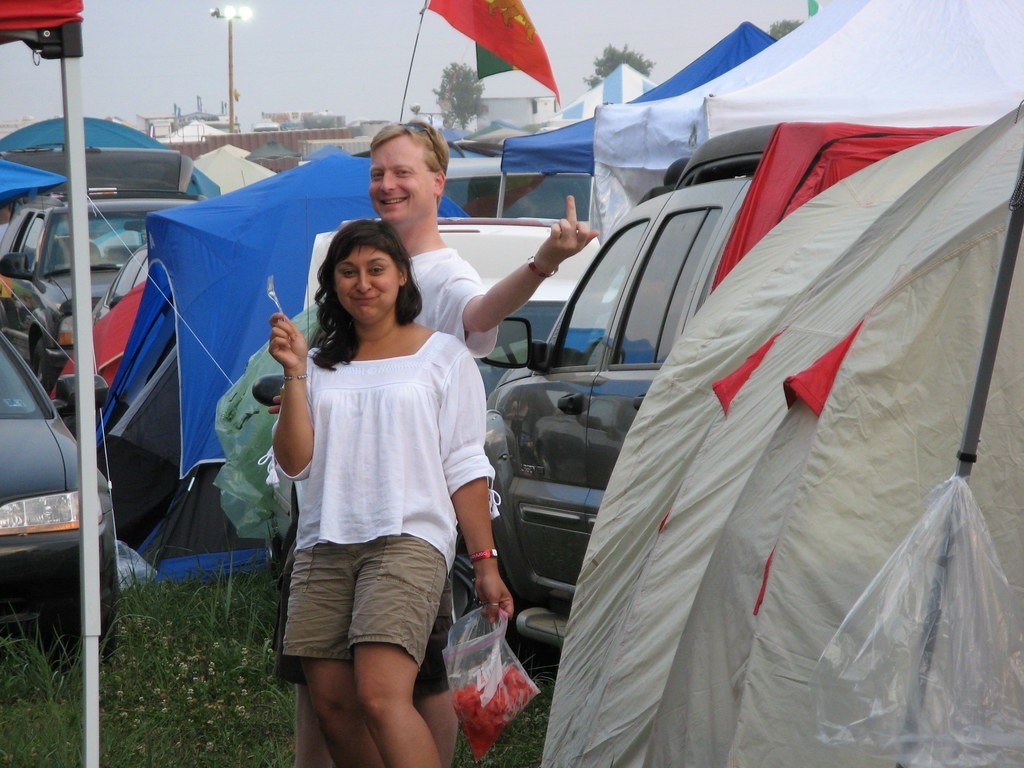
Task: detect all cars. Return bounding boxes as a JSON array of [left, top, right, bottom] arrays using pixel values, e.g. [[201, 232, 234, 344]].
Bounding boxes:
[[0, 120, 985, 692]]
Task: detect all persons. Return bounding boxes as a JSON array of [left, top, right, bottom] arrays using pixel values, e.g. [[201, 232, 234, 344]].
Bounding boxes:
[[0, 204, 13, 246], [295, 120, 603, 768], [270, 219, 514, 768]]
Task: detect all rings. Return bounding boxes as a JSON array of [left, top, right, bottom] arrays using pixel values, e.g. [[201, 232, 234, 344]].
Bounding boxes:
[[489, 602, 498, 606]]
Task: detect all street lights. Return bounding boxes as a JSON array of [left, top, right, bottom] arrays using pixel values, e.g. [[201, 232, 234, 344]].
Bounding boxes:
[[211, 5, 255, 133]]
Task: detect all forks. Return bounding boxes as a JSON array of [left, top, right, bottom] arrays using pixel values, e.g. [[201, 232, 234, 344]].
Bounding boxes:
[[266, 275, 286, 323]]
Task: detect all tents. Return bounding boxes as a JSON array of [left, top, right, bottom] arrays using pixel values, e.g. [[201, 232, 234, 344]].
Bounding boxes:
[[541, 103, 1024, 767], [450, 2, 1024, 220], [106, 142, 472, 592], [1, 114, 219, 244]]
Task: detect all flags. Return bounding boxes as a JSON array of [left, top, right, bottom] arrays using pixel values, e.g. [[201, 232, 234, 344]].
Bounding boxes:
[[427, 0, 561, 101], [0, 151, 67, 200]]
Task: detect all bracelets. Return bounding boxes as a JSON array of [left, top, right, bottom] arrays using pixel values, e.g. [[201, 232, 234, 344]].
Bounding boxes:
[[469, 548, 498, 563], [528, 256, 558, 277], [285, 375, 308, 381]]
